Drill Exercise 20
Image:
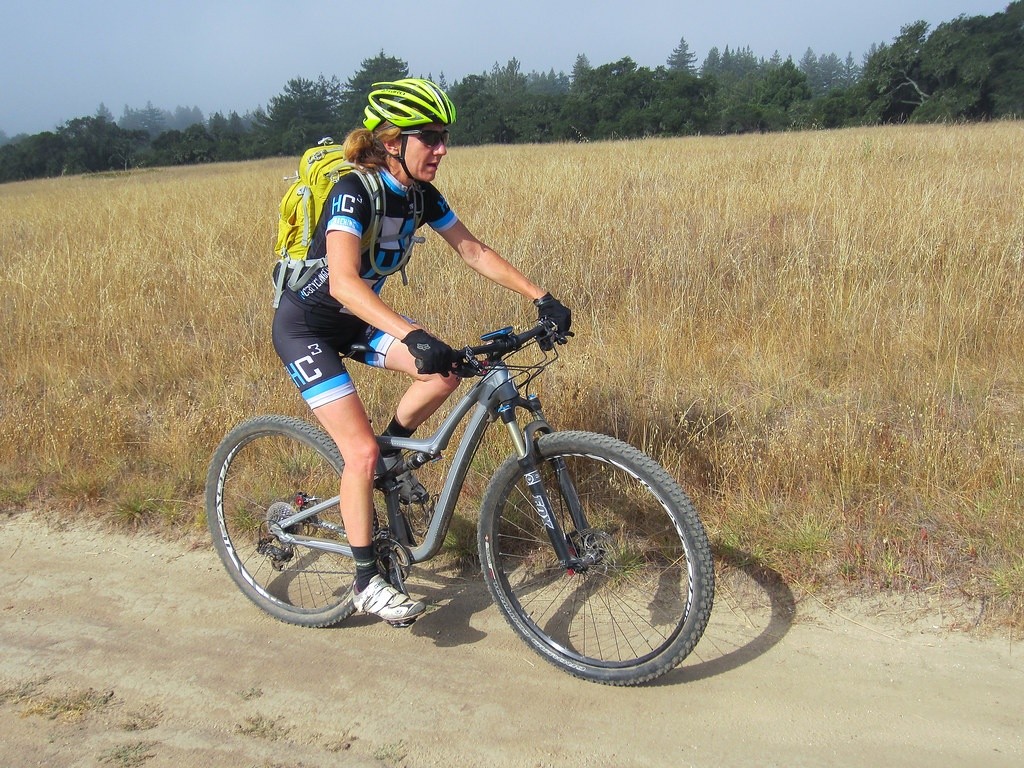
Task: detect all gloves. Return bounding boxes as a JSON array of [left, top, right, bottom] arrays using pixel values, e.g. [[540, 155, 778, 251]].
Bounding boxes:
[[533, 291, 571, 341], [400, 329, 463, 377]]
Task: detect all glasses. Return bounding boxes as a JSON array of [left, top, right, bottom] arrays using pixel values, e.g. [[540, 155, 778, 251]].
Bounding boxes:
[[400, 130, 450, 148]]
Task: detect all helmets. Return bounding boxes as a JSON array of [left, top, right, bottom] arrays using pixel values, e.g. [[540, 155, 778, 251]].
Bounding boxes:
[[362, 78, 457, 132]]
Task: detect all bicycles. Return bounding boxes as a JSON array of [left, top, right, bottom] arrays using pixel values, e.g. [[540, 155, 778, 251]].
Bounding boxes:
[[206, 315, 715, 688]]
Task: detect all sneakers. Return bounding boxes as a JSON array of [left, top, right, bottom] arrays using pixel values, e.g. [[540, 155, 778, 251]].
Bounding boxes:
[[353, 574, 428, 621], [372, 452, 430, 504]]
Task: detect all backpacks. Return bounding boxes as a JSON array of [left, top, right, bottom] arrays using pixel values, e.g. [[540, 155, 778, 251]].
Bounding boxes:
[[274, 137, 424, 268]]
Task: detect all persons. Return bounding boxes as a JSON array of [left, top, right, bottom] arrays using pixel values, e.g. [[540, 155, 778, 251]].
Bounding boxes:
[[267, 78, 574, 623]]
